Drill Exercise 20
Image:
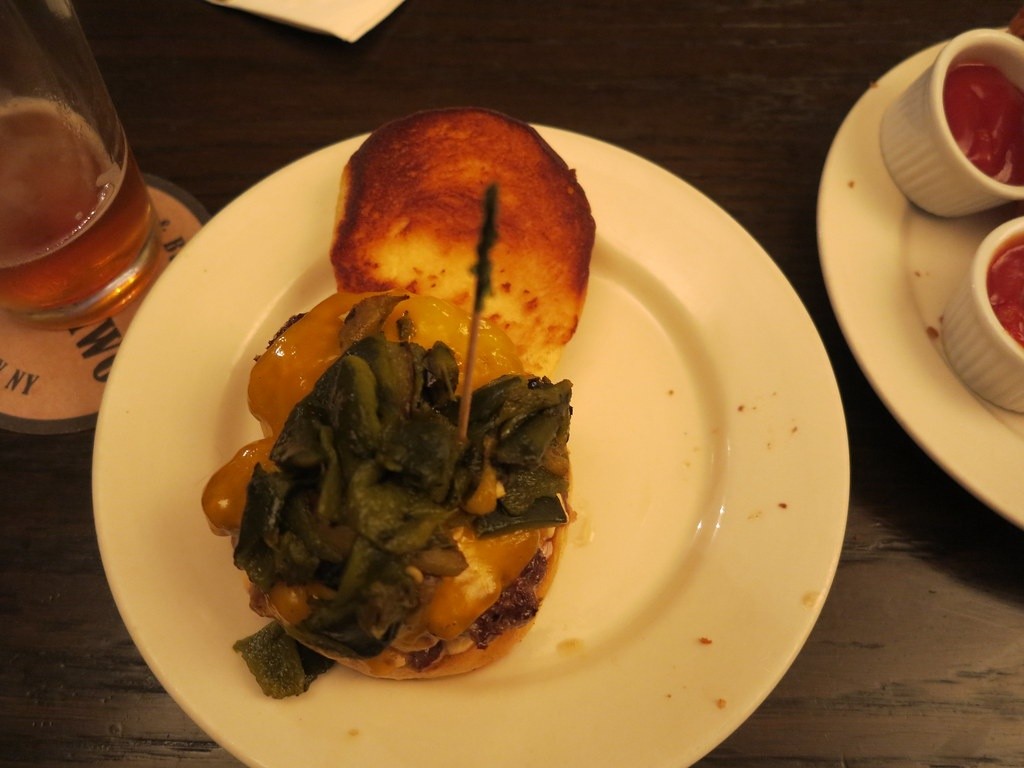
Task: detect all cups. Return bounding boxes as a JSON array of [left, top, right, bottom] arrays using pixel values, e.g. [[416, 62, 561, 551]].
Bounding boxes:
[[943, 218, 1024, 415], [881, 27, 1024, 217], [0, 0, 165, 330]]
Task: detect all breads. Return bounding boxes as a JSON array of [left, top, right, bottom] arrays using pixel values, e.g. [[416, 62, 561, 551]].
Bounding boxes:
[[258, 102, 598, 683]]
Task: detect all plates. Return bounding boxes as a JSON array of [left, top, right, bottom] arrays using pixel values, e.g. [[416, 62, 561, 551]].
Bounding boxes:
[[91, 123, 849, 768], [818, 25, 1024, 528]]
